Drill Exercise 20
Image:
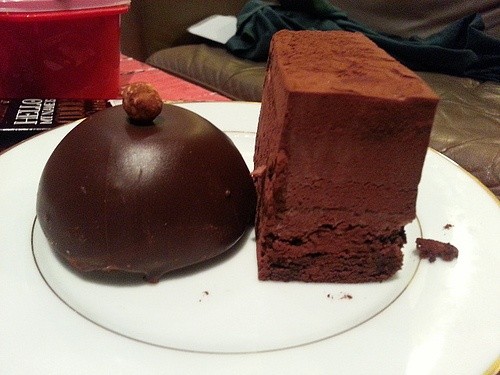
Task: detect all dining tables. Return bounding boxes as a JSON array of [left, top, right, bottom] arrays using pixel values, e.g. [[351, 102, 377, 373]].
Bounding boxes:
[[0, 100, 500, 374]]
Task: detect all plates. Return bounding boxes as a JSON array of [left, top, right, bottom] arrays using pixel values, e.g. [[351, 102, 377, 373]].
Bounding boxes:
[[0, 100, 499, 373]]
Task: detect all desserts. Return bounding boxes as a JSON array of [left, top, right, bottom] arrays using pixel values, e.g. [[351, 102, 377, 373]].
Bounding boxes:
[[249, 30, 439, 284], [36, 81, 258, 280]]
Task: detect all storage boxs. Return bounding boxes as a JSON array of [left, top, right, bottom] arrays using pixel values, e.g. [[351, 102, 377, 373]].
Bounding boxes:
[[0, 0, 129, 100]]
